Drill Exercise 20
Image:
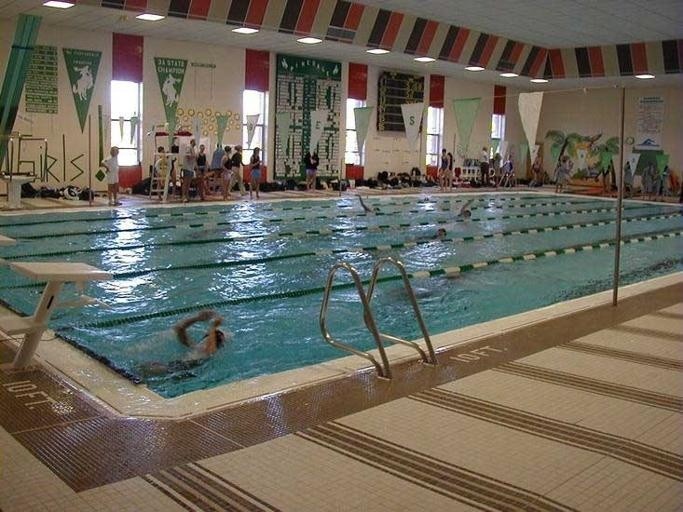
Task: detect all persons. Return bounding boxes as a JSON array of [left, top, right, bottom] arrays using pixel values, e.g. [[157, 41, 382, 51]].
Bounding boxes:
[[480, 145, 494, 186], [639, 163, 672, 200], [433, 228, 448, 239], [528, 157, 541, 188], [100, 146, 123, 205], [438, 149, 450, 192], [304, 151, 319, 192], [495, 154, 514, 186], [153, 140, 263, 204], [356, 193, 381, 213], [133, 310, 227, 378], [553, 154, 574, 192], [445, 152, 454, 191], [458, 197, 476, 218]]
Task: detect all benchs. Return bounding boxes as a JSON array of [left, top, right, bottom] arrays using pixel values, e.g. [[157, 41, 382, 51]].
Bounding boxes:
[[451, 166, 498, 189]]
